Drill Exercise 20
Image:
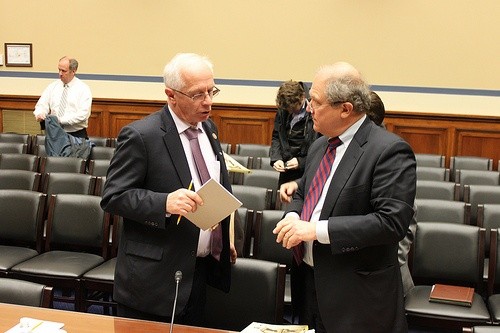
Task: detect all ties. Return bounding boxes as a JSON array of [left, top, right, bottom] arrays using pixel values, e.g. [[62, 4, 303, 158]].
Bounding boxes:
[[58, 84, 68, 117], [293, 135, 343, 264], [183, 127, 223, 263]]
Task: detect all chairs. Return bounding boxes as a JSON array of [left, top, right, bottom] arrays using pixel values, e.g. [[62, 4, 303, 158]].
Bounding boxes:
[[0, 131, 500, 333]]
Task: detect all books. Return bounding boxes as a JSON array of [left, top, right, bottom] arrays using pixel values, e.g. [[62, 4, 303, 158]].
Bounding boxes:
[[178, 176, 244, 232], [238, 320, 317, 333], [427, 281, 477, 308]]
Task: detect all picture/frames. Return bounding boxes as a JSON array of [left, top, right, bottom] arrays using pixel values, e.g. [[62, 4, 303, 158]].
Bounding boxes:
[[4, 43, 34, 67]]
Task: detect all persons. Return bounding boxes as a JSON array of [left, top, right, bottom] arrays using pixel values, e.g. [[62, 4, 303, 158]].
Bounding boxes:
[[279, 90, 418, 300], [98, 53, 235, 328], [33, 55, 93, 140], [272, 59, 418, 333], [269, 80, 318, 190]]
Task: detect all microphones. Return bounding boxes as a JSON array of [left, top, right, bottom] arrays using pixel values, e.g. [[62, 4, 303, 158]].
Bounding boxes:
[[169, 270, 183, 333]]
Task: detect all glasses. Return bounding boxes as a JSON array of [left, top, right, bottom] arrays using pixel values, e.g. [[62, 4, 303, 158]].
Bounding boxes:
[[304, 95, 347, 112], [167, 85, 220, 103]]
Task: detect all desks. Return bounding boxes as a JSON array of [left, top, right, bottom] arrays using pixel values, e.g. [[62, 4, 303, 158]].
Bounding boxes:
[[0, 300, 236, 333]]
